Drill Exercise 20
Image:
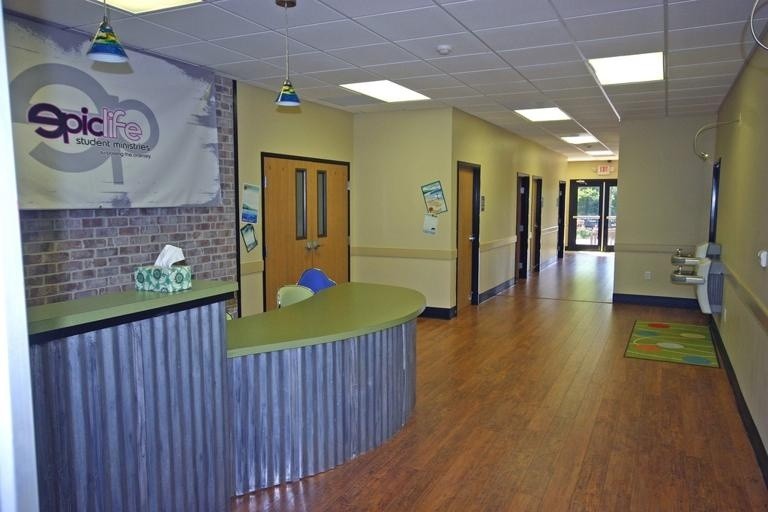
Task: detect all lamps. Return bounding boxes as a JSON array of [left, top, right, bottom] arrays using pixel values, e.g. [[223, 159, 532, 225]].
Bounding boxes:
[[86, 0, 129, 63], [273, 0, 301, 106]]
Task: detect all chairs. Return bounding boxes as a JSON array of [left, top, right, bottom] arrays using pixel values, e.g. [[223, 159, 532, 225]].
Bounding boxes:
[[276, 267, 337, 309]]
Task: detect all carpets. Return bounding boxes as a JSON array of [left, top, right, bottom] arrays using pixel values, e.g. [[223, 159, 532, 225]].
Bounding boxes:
[[624, 320, 720, 368]]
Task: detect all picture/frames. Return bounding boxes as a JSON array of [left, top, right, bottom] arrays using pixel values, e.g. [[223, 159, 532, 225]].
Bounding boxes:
[[240, 183, 259, 224], [420, 180, 448, 214], [240, 224, 258, 253]]
